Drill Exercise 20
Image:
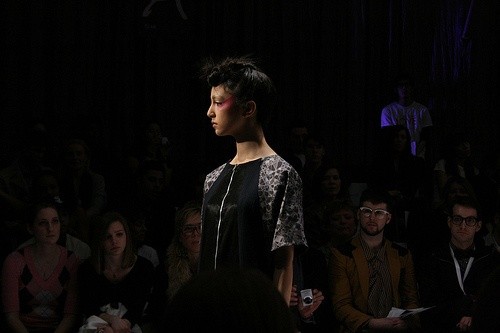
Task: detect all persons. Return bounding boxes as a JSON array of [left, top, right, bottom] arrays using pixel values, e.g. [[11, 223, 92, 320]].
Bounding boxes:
[[197, 53, 309, 310], [0, 77, 500, 333]]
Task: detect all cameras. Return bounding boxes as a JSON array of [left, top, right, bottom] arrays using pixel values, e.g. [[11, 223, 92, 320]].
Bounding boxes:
[[301, 289, 313, 307]]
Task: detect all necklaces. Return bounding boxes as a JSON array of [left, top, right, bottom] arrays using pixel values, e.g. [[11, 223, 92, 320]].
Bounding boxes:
[[448, 243, 477, 296], [105, 264, 121, 279]]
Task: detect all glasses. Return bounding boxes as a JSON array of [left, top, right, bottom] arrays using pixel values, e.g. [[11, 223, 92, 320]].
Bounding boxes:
[[360, 206, 389, 220], [451, 214, 476, 228], [181, 223, 202, 237]]
